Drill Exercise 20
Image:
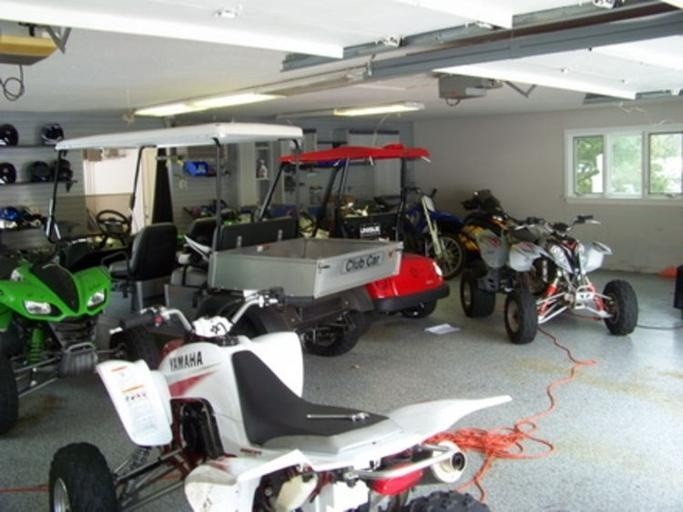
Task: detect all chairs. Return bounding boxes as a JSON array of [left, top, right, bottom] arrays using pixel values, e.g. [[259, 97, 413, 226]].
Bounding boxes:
[[101, 223, 178, 281], [190, 216, 223, 239]]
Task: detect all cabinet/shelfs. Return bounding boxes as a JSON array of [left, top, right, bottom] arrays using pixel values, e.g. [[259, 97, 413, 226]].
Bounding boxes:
[[252, 141, 273, 206], [0, 144, 69, 231]]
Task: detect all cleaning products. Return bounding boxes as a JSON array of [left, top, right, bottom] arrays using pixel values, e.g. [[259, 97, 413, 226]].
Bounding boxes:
[[257, 160, 268, 179]]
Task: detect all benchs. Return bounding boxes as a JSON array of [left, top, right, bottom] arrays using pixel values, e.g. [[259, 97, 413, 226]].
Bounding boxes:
[[171, 217, 292, 287]]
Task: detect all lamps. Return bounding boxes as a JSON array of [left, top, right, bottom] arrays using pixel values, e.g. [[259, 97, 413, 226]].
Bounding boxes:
[[333, 101, 426, 118], [581, 87, 677, 106], [135, 89, 285, 118]]
